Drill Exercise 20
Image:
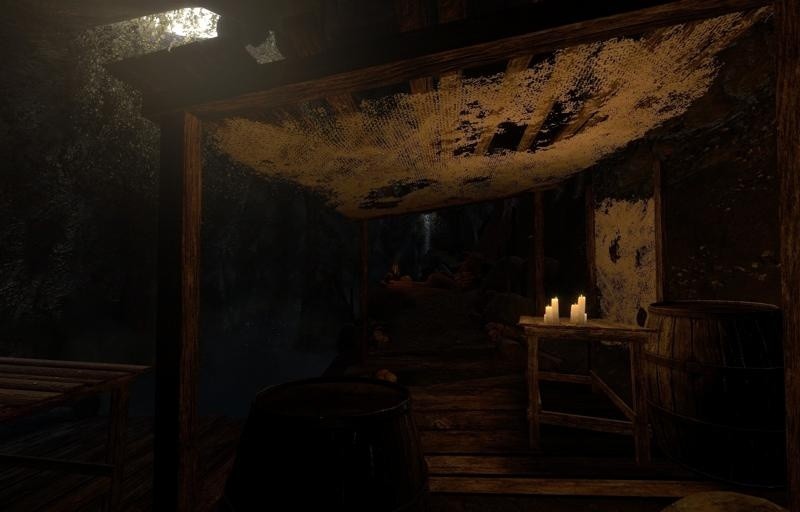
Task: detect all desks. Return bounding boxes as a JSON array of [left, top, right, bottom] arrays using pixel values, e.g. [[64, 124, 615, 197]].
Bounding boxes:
[[517, 313, 662, 473]]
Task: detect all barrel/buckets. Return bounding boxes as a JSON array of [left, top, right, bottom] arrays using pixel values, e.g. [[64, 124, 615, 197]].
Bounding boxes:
[[227, 376, 426, 512], [637, 297, 782, 479]]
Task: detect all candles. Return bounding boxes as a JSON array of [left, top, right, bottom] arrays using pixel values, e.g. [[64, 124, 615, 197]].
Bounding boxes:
[[542, 293, 589, 324]]
